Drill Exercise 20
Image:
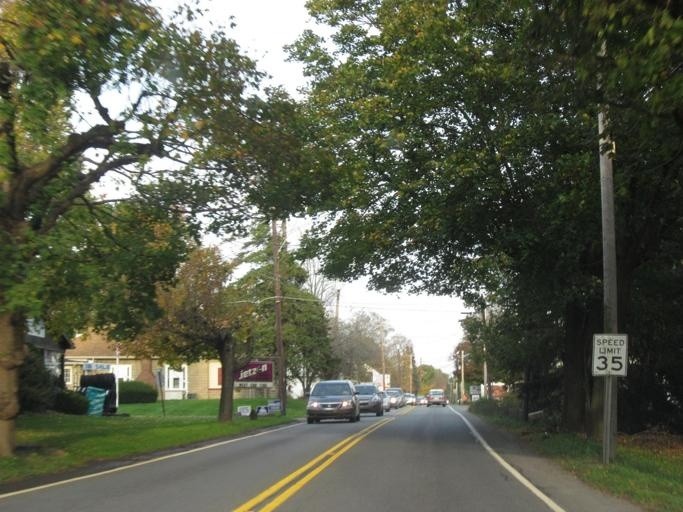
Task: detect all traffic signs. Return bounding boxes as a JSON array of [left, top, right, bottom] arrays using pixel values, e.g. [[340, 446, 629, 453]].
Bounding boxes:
[[592, 333, 627, 377]]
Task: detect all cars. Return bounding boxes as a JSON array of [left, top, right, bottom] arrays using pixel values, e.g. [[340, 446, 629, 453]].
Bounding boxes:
[[353, 382, 384, 417], [304, 379, 362, 424], [427, 389, 446, 407], [380, 388, 428, 413]]
